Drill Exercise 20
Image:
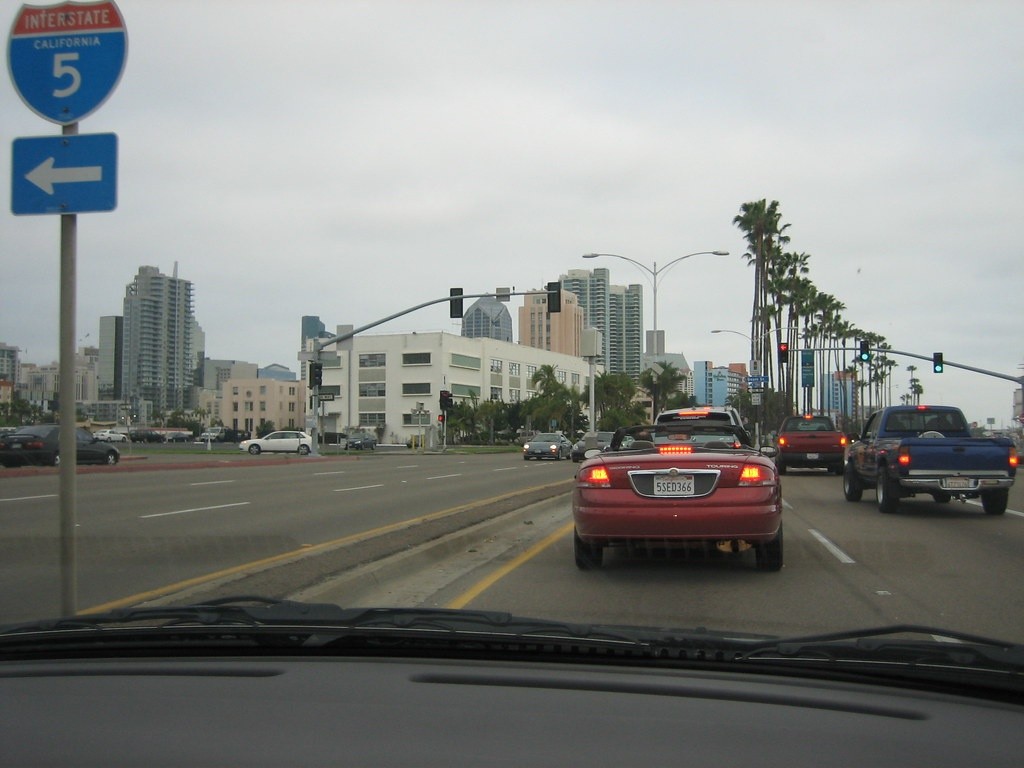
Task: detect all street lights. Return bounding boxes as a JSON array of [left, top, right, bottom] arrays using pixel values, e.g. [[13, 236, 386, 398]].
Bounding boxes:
[[710, 326, 802, 450], [582, 250, 730, 434]]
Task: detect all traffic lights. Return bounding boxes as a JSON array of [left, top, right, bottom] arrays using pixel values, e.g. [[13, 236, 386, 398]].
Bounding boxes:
[[779, 343, 788, 365], [440, 390, 453, 410], [933, 352, 943, 374], [860, 341, 869, 362], [438, 415, 443, 421], [309, 362, 322, 390]]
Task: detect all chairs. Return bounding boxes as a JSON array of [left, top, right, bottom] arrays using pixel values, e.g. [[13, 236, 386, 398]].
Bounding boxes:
[[704, 441, 730, 449], [629, 440, 654, 449]]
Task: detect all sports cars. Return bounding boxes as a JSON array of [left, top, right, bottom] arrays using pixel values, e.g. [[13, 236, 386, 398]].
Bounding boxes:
[[572, 424, 786, 575]]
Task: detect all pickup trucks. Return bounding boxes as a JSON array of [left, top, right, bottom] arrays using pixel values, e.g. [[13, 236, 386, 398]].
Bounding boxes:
[[775, 413, 845, 475], [841, 405, 1019, 514]]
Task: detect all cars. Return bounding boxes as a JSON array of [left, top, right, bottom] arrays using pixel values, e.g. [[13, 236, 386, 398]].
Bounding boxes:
[[522, 433, 573, 459], [653, 408, 746, 448], [129, 430, 166, 443], [346, 432, 377, 451], [1, 423, 119, 469], [198, 427, 231, 442], [239, 431, 320, 455], [571, 432, 617, 462], [91, 430, 126, 442], [165, 431, 189, 442]]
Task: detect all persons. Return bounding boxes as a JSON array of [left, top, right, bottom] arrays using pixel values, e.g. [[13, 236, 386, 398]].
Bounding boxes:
[[634, 430, 653, 442], [792, 422, 799, 431]]
